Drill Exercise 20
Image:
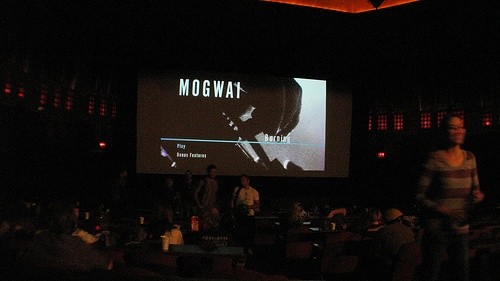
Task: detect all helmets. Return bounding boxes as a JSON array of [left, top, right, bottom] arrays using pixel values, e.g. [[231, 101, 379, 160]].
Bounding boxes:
[[240, 204, 248, 213]]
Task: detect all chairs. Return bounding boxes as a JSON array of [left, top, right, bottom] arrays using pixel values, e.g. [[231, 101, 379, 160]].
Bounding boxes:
[[104, 219, 500, 281]]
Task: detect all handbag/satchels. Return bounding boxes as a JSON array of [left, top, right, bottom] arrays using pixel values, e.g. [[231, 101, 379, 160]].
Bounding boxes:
[[196, 179, 207, 195]]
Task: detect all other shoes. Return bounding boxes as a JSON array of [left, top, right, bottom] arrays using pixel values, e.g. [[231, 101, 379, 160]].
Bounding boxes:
[[248, 249, 253, 255]]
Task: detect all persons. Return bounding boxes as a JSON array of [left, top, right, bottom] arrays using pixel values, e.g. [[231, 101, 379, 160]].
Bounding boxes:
[[229, 173, 259, 256], [278, 202, 307, 244], [173, 164, 220, 234], [404, 114, 485, 281], [368, 207, 417, 281], [0, 188, 119, 281]]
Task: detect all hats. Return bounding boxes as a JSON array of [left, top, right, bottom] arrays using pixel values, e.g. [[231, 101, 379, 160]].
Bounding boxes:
[[384, 208, 403, 222]]
